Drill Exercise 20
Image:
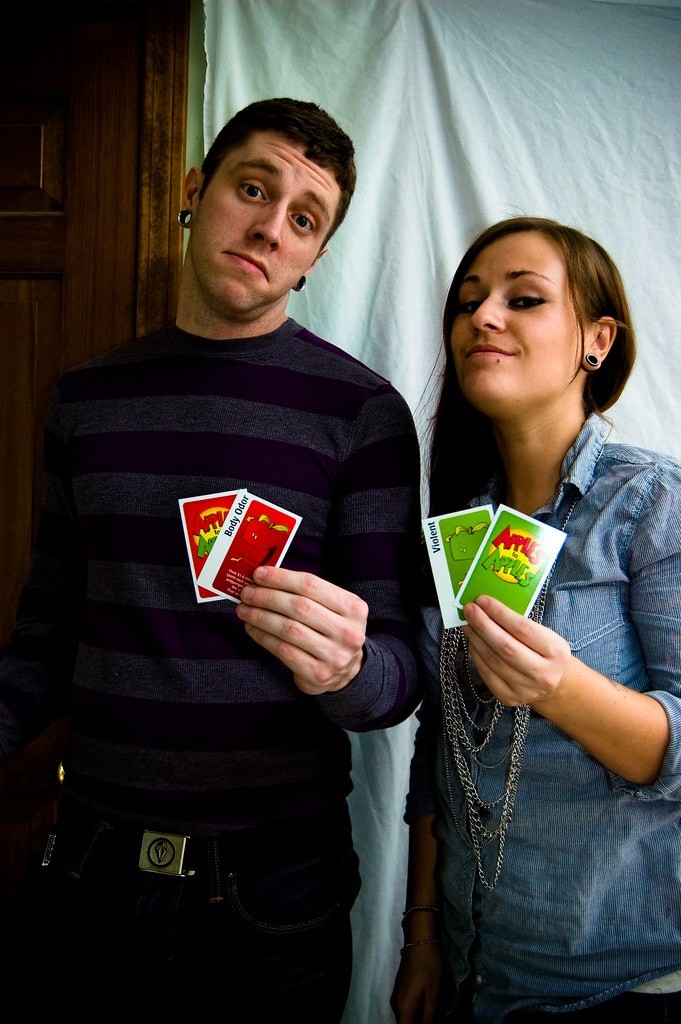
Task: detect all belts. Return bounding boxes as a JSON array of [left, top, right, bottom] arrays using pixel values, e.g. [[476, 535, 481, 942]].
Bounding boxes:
[[9, 799, 352, 881]]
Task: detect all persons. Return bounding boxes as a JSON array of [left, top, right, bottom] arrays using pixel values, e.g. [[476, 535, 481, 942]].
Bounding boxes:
[[390, 215, 681, 1024], [0, 97, 431, 1023]]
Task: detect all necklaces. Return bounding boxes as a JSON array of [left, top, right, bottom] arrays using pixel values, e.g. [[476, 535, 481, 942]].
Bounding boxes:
[[439, 488, 584, 889]]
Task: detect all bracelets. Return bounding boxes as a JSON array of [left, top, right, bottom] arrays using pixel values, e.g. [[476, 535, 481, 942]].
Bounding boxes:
[[399, 905, 436, 952]]
